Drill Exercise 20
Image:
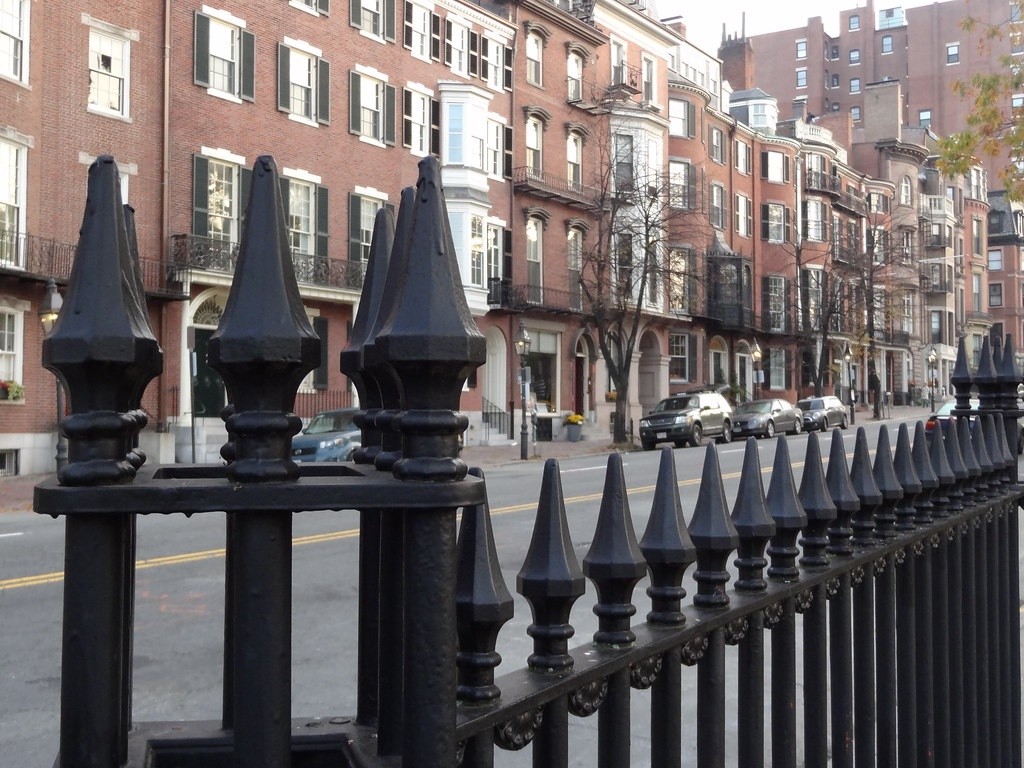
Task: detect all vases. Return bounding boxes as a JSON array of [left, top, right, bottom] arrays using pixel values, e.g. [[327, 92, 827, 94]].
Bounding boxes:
[[0, 387, 8, 399], [567, 423, 584, 442]]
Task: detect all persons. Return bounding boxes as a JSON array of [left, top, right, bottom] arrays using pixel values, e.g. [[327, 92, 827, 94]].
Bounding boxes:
[[868, 370, 876, 405], [921, 382, 929, 408]]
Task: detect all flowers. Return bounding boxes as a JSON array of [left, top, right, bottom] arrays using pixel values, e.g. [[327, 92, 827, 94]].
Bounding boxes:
[[0, 379, 25, 402], [563, 415, 585, 427]]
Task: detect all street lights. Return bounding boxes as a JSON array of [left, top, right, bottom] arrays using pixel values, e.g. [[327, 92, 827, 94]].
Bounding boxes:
[[511, 321, 534, 461], [750, 336, 766, 403], [843, 340, 857, 427], [928, 346, 941, 413], [37, 275, 71, 475]]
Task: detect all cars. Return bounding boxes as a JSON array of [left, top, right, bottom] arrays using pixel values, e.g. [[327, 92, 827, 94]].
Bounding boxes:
[[795, 396, 850, 434], [729, 397, 805, 440], [925, 397, 1023, 456], [291, 405, 364, 461]]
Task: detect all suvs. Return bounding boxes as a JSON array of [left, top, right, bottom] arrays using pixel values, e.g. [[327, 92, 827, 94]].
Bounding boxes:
[[638, 382, 734, 451]]
